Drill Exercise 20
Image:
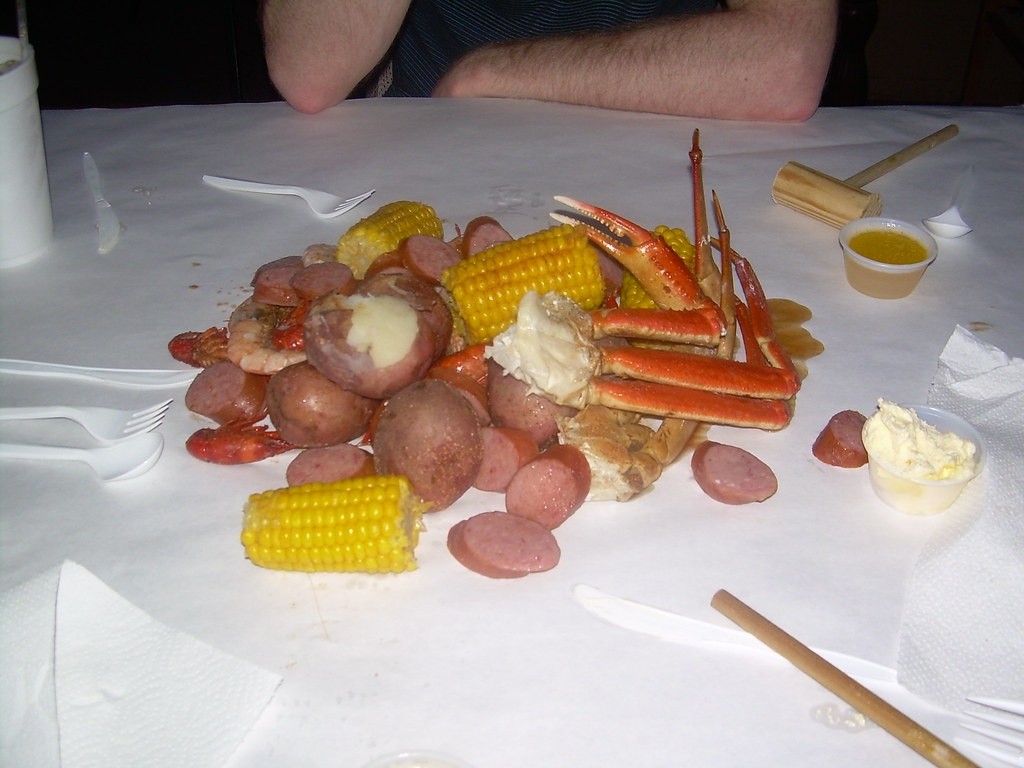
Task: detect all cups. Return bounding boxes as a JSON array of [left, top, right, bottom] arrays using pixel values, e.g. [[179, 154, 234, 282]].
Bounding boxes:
[[0, 37, 53, 268]]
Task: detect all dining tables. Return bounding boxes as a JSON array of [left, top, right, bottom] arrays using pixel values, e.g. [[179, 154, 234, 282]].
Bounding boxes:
[[0, 98, 1024, 768]]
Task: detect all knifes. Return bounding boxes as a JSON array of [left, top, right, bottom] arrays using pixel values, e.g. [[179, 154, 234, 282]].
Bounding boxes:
[[0, 360, 204, 386], [585, 585, 956, 715], [84, 152, 120, 252]]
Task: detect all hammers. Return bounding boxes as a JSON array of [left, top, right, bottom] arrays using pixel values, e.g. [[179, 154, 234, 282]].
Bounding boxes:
[[771, 118, 959, 231]]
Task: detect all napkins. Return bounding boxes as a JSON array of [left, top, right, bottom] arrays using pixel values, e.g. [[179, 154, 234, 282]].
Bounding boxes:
[[895, 323, 1024, 738], [0, 559, 286, 768]]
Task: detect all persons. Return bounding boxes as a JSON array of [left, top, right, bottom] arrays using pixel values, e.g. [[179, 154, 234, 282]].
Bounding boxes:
[[260, 0, 838, 123]]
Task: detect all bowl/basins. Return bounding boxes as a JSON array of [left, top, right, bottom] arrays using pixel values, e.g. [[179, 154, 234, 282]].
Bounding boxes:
[[839, 217, 937, 300], [862, 405, 987, 517]]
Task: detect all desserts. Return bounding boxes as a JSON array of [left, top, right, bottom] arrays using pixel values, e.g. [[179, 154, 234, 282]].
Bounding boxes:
[[865, 399, 978, 514]]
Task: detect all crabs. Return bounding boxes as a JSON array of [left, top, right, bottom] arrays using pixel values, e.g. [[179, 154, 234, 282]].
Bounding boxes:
[[483, 128, 804, 430]]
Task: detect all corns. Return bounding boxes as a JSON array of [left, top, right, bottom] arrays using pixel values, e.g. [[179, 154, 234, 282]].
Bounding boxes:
[[336, 200, 445, 279], [242, 476, 423, 573], [622, 226, 700, 351], [440, 222, 609, 342]]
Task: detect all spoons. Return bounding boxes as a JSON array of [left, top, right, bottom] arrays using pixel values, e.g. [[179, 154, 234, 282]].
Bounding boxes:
[[0, 433, 163, 481]]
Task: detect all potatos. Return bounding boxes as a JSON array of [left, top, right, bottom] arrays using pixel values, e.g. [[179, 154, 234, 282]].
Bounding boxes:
[[269, 270, 577, 503]]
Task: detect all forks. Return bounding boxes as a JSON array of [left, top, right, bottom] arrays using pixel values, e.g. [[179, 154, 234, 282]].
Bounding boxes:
[[203, 174, 376, 218], [0, 397, 174, 441], [954, 695, 1024, 768]]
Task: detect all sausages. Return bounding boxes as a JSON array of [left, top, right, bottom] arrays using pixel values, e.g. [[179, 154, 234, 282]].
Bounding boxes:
[[461, 214, 514, 259], [473, 428, 590, 523], [185, 360, 266, 425], [447, 510, 561, 579], [285, 443, 373, 485], [255, 255, 354, 306], [692, 439, 778, 507], [813, 410, 869, 468]]
